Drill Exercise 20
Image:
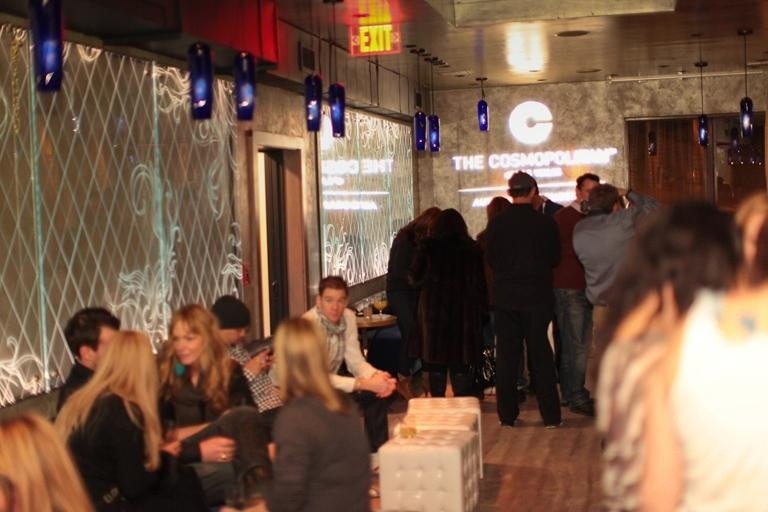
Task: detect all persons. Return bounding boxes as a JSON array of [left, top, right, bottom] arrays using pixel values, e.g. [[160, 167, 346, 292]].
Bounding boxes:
[[574, 185, 659, 417], [210, 295, 283, 413], [419, 208, 487, 407], [265, 317, 371, 512], [2, 411, 92, 512], [302, 277, 396, 499], [57, 308, 270, 512], [384, 209, 444, 398], [556, 173, 599, 404], [474, 195, 513, 396], [489, 172, 562, 428], [52, 330, 207, 511], [159, 306, 274, 464]]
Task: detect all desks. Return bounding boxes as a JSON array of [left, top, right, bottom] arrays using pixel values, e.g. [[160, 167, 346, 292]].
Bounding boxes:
[[356, 311, 398, 368]]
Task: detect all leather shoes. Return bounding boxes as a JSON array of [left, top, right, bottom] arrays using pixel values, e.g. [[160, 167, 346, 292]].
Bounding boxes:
[[570, 402, 596, 416]]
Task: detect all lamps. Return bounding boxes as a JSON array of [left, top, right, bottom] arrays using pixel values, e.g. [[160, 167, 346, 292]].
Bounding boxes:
[[410, 48, 425, 152], [32, 1, 63, 96], [323, 0, 345, 138], [189, 42, 215, 121], [694, 62, 710, 147], [303, 1, 323, 132], [233, 49, 257, 123], [736, 29, 754, 143], [425, 57, 440, 153], [476, 78, 489, 132]]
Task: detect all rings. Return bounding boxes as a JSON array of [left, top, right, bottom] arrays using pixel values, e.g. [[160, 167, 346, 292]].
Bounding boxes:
[[219, 454, 224, 459]]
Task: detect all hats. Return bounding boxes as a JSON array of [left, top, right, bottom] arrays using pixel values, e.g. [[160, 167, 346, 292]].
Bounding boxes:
[[211, 294, 251, 330], [507, 169, 537, 190]]
